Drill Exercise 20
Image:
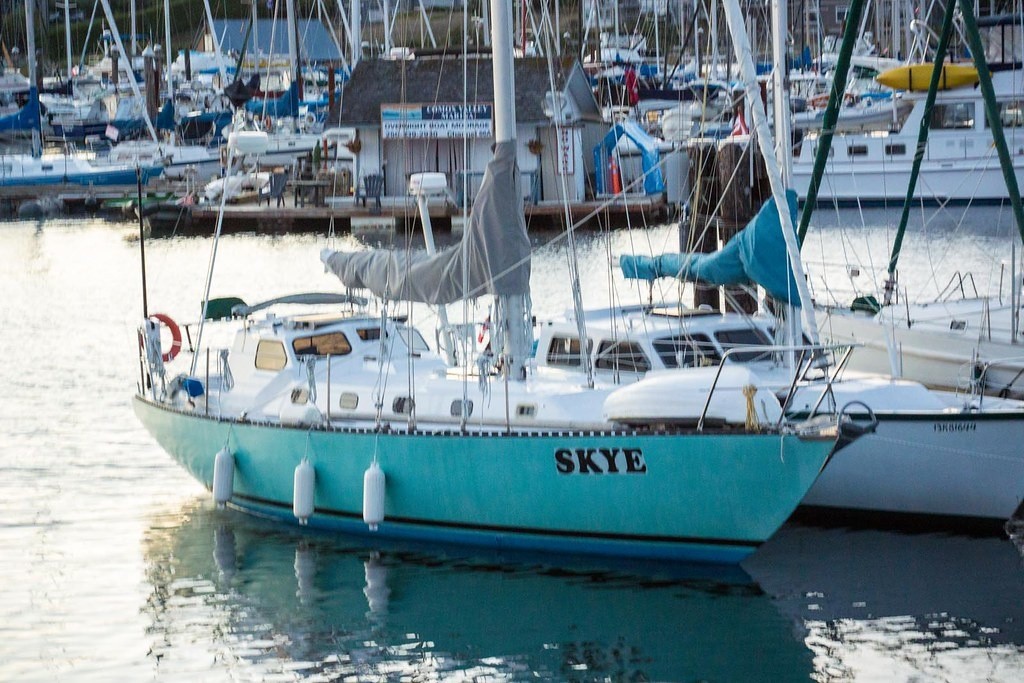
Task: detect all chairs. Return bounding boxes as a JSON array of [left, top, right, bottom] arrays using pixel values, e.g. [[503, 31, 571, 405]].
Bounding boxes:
[[255, 172, 288, 211], [361, 173, 385, 211]]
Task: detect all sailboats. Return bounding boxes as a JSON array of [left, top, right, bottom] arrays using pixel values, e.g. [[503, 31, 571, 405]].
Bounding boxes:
[[0, 0, 1024, 238], [129, 0, 876, 587], [601, 0, 1024, 539]]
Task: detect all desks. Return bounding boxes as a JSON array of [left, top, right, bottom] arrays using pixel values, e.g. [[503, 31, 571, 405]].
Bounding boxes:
[[285, 179, 332, 209]]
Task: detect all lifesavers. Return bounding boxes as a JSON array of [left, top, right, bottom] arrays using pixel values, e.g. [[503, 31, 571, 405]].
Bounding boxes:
[[140, 313, 182, 362]]
